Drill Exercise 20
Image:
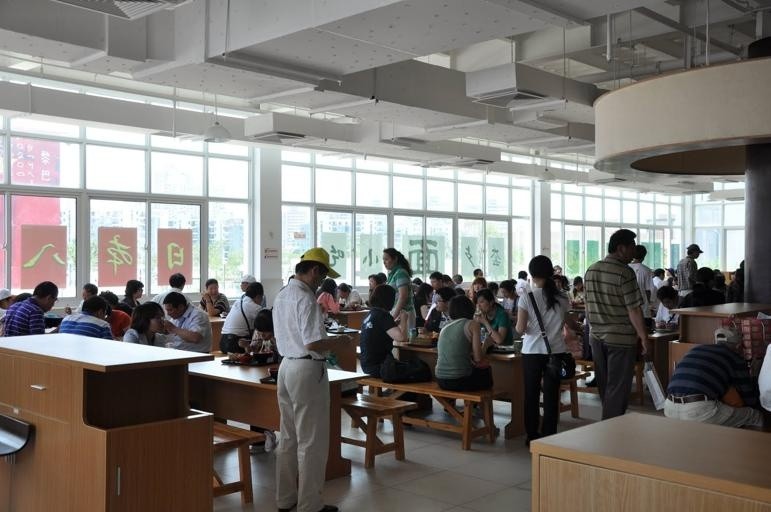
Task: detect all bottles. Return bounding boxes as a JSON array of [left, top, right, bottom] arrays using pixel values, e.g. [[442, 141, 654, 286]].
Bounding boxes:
[[409, 328, 418, 343], [480, 328, 485, 343]]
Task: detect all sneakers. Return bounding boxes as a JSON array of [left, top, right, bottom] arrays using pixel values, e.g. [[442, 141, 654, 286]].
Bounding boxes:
[[472, 406, 483, 417], [443, 406, 463, 417], [318, 505, 338, 512], [264, 431, 276, 452]]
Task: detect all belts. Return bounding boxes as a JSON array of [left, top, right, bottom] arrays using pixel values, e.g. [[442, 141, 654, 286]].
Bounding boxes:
[[668, 394, 712, 403], [288, 355, 326, 361]]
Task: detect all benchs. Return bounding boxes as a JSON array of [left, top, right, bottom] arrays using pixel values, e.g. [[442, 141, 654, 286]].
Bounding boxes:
[[213, 421, 266, 505]]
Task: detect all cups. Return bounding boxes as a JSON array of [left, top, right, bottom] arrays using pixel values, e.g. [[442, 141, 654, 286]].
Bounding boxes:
[[337, 326, 345, 331], [513, 340, 523, 355]]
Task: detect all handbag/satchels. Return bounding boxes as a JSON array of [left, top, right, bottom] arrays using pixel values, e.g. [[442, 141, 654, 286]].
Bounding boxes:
[[380, 354, 431, 383], [643, 361, 666, 411], [718, 313, 771, 378], [549, 353, 576, 379]]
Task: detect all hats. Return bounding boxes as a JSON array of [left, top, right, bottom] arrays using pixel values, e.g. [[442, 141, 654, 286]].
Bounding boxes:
[[300, 248, 341, 278], [686, 244, 703, 253], [666, 268, 674, 273], [0, 288, 12, 301], [242, 274, 256, 284]]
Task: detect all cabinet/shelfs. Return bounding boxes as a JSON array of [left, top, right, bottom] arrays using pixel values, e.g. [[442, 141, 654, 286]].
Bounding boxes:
[[668, 302, 771, 407], [0, 332, 215, 511], [529, 411, 771, 512]]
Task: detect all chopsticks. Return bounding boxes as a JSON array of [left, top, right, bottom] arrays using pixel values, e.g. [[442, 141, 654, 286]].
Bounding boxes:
[[232, 354, 241, 360]]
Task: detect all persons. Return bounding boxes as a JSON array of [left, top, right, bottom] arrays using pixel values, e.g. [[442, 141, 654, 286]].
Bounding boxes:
[[270, 247, 356, 512], [0, 272, 280, 448], [315, 230, 771, 449]]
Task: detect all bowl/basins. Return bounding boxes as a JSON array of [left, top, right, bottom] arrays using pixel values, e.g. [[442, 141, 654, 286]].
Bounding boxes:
[[250, 352, 273, 363], [416, 336, 437, 345], [269, 367, 279, 380]]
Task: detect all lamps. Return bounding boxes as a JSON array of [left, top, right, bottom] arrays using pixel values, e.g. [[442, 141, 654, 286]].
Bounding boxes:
[[204, 95, 232, 143]]
